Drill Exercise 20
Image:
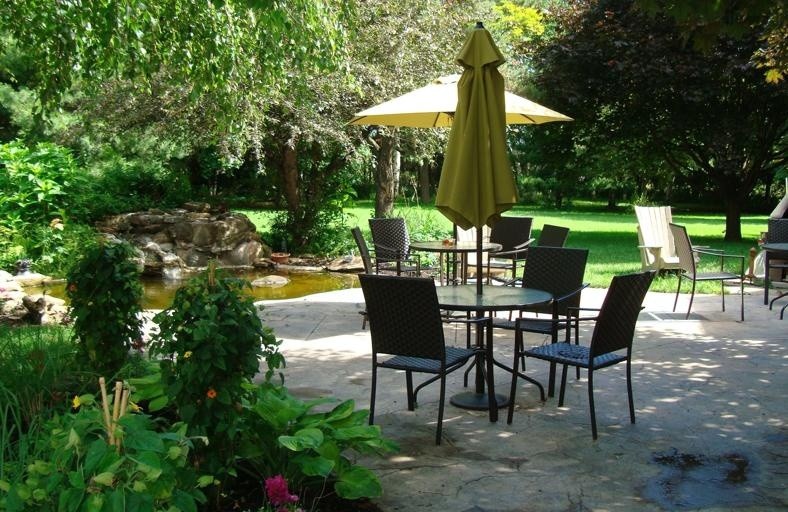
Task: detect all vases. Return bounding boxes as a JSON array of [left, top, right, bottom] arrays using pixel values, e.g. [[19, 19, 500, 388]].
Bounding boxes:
[[271, 252, 290, 264]]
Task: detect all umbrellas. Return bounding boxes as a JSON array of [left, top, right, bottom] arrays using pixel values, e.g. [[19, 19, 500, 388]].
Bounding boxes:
[[345, 71, 578, 244], [434, 21, 512, 292]]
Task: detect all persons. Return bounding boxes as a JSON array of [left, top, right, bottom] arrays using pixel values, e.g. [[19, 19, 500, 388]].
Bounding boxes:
[[763, 188, 788, 282]]
